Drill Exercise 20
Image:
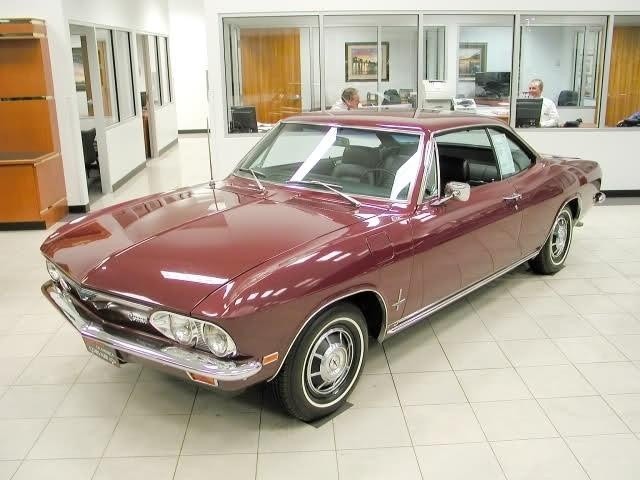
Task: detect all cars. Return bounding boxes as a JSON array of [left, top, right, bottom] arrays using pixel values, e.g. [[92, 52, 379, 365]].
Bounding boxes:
[[40, 116, 606, 423]]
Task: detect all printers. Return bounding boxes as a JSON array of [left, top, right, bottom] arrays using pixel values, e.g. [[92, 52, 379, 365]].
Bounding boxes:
[[422, 79, 452, 112]]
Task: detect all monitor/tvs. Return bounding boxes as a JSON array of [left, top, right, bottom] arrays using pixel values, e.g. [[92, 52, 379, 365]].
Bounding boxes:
[[475, 72, 510, 101], [510, 99, 543, 127], [231, 107, 258, 133]]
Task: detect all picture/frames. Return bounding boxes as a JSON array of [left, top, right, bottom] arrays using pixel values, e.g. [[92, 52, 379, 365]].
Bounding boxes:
[[458, 42, 488, 81], [345, 42, 389, 82]]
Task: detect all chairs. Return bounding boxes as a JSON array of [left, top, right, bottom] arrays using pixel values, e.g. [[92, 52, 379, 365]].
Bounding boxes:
[[438, 155, 464, 195], [558, 89, 578, 105], [332, 144, 382, 185], [81, 127, 101, 178], [379, 153, 411, 194], [463, 159, 499, 186]]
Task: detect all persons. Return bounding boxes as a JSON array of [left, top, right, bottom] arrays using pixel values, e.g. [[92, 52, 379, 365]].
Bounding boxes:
[[528, 77, 561, 127], [330, 86, 363, 110]]
